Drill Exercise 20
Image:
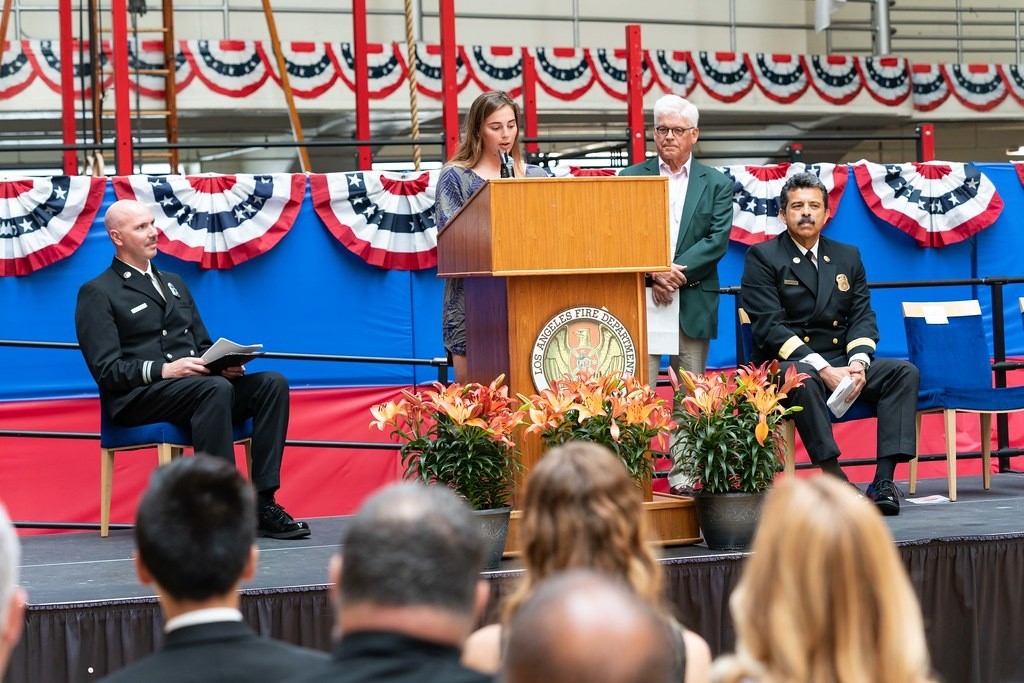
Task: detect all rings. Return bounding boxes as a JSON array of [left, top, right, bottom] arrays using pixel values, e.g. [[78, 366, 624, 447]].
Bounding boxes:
[[237, 372, 240, 376]]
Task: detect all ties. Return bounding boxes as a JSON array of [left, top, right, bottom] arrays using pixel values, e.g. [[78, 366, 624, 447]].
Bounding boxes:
[[805, 250, 817, 272], [145, 272, 152, 283]]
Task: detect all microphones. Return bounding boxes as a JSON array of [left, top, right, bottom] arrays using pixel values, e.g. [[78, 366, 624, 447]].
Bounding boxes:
[[506, 156, 515, 178]]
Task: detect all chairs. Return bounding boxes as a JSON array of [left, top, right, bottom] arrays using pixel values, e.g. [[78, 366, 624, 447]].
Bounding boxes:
[[100, 387, 254, 538], [736, 294, 1024, 502]]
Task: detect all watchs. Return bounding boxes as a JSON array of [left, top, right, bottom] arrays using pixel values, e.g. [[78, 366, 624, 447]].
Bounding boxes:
[[855, 359, 867, 370]]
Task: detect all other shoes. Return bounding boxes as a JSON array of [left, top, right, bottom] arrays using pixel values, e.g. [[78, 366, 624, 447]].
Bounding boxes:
[[670, 485, 695, 496]]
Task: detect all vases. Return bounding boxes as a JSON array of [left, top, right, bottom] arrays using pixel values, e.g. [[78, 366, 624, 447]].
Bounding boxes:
[[465, 502, 510, 573], [690, 486, 766, 552]]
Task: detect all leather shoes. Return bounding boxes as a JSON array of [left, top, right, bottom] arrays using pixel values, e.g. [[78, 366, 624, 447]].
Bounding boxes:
[[865, 479, 905, 516], [254, 503, 312, 539]]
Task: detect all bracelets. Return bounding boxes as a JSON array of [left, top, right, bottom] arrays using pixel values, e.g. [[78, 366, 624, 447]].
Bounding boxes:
[[650, 273, 656, 280], [162, 363, 167, 378]]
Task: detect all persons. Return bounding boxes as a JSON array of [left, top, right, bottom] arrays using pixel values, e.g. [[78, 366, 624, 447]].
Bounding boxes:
[[618, 95, 734, 498], [504, 563, 686, 683], [739, 172, 920, 515], [74, 196, 311, 538], [92, 453, 337, 683], [313, 476, 497, 683], [709, 468, 946, 683], [434, 91, 548, 391], [0, 502, 24, 683], [458, 439, 715, 683]]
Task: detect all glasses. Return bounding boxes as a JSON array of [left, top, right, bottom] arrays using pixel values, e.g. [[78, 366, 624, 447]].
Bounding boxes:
[[655, 125, 695, 137]]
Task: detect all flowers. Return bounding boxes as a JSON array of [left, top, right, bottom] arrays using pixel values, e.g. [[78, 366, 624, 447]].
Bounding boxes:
[[365, 371, 531, 523], [518, 370, 672, 478], [663, 355, 814, 494]]
